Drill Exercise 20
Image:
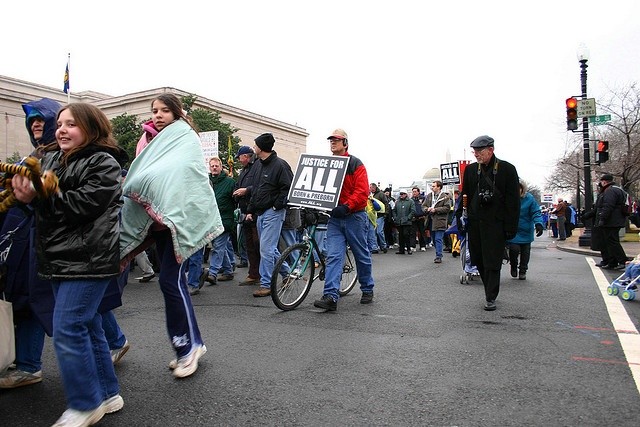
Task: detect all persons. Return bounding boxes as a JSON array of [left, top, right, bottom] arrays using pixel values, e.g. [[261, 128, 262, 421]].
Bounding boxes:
[[245, 133, 295, 297], [208, 157, 238, 285], [505, 178, 543, 280], [235, 146, 261, 285], [594, 174, 628, 270], [282, 206, 302, 279], [312, 222, 326, 268], [119, 94, 225, 378], [581, 182, 617, 266], [541, 198, 579, 241], [186, 248, 209, 295], [0, 97, 131, 389], [236, 222, 248, 268], [365, 179, 463, 264], [314, 129, 375, 310], [134, 250, 156, 283], [455, 135, 521, 311], [633, 197, 640, 213], [218, 233, 235, 273], [12, 104, 129, 427]]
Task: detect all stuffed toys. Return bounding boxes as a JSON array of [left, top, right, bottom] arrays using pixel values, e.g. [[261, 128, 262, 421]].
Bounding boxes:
[[0, 155, 59, 213]]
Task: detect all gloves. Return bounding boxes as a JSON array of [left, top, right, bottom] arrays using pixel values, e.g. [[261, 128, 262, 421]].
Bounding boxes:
[[332, 203, 349, 217], [506, 227, 516, 240], [535, 223, 545, 236], [580, 212, 587, 223], [456, 216, 463, 231], [598, 219, 604, 226]]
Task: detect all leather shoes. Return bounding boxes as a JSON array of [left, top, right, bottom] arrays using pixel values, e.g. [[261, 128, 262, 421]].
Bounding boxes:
[[595, 261, 606, 267], [138, 273, 156, 282], [601, 264, 615, 269], [557, 238, 564, 241], [613, 263, 626, 269], [281, 275, 291, 289], [252, 286, 271, 297], [238, 278, 260, 285], [434, 257, 442, 263], [372, 250, 379, 254], [206, 273, 217, 283], [395, 251, 405, 254], [218, 273, 235, 280], [510, 265, 518, 277], [519, 272, 527, 280], [314, 294, 337, 309], [443, 245, 451, 250], [236, 263, 248, 267], [483, 298, 496, 309], [383, 247, 387, 253], [360, 291, 373, 303], [408, 249, 412, 254]]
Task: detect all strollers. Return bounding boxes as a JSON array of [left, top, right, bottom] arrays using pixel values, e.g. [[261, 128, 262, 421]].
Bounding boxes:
[[459, 234, 481, 285], [607, 254, 640, 302]]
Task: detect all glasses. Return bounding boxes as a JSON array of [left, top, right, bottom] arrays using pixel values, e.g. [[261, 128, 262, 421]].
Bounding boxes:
[[328, 137, 341, 143], [400, 193, 406, 195], [471, 148, 486, 152]]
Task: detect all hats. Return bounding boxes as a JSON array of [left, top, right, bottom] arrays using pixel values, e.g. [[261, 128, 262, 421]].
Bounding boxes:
[[383, 187, 390, 193], [470, 135, 494, 148], [26, 105, 45, 119], [600, 174, 613, 181], [234, 145, 254, 159], [254, 132, 275, 153], [326, 128, 348, 141]]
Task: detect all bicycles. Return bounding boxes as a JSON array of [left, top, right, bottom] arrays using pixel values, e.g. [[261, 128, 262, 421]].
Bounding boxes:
[[270, 207, 359, 312]]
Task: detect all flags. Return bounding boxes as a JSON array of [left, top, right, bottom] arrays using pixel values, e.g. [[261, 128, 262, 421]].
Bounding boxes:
[[63, 63, 69, 94]]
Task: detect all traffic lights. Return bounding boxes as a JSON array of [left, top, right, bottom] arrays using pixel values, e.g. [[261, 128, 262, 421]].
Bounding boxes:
[[599, 140, 609, 163], [566, 98, 577, 130]]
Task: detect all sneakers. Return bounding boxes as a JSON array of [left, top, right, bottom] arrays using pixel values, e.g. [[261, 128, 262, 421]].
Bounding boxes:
[[110, 338, 130, 366], [1, 366, 43, 388], [313, 260, 320, 268], [172, 341, 208, 379], [168, 351, 179, 370], [412, 248, 416, 252], [292, 267, 301, 275], [187, 286, 201, 296], [48, 402, 105, 427], [421, 247, 427, 252], [199, 267, 210, 289], [98, 394, 125, 415]]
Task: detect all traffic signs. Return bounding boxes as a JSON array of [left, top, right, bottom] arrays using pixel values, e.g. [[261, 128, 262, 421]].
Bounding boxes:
[[590, 114, 611, 121]]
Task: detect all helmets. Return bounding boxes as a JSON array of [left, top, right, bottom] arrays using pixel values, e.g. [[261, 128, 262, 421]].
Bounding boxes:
[[400, 188, 408, 195], [558, 198, 563, 202]]
